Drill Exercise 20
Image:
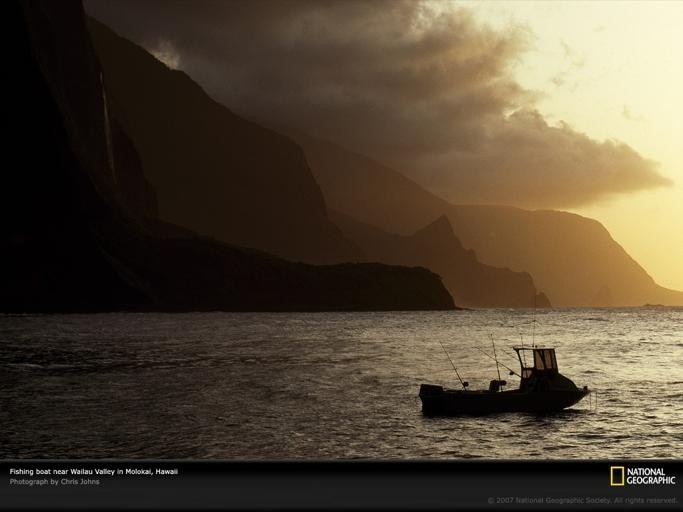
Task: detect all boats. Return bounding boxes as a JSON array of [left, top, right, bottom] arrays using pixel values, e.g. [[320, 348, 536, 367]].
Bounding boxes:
[[418, 289, 593, 415]]
